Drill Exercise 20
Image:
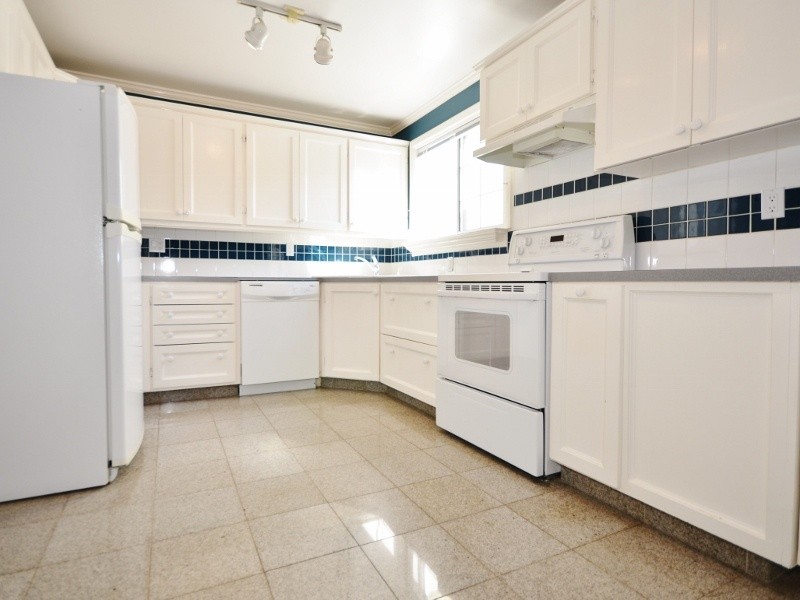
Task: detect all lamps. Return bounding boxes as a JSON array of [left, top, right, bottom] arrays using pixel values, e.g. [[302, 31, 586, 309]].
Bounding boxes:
[[245, 8, 266, 48], [314, 24, 333, 65]]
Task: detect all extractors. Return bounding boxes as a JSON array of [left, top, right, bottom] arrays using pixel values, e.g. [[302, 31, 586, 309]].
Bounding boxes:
[[473, 103, 595, 168]]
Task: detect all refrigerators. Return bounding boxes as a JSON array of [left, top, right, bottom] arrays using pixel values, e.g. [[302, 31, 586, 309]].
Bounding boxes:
[[0, 72, 144, 505]]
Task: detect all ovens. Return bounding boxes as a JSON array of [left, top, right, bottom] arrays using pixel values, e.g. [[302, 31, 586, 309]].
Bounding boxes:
[[434, 284, 561, 477]]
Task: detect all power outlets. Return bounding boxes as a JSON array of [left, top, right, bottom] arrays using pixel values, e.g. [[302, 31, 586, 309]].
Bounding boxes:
[[761, 187, 785, 221]]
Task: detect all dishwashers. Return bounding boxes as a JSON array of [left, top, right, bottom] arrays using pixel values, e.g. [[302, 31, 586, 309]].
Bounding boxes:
[[239, 280, 321, 398]]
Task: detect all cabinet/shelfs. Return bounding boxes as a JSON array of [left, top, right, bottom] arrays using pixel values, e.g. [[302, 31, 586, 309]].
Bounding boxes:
[[151, 282, 242, 404], [127, 96, 409, 240], [380, 281, 438, 418], [550, 277, 800, 585], [594, 0, 800, 179], [320, 281, 387, 393], [474, 0, 596, 169]]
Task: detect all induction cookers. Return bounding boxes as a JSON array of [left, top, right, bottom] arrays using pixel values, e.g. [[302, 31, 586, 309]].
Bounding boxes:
[[438, 214, 636, 282]]
